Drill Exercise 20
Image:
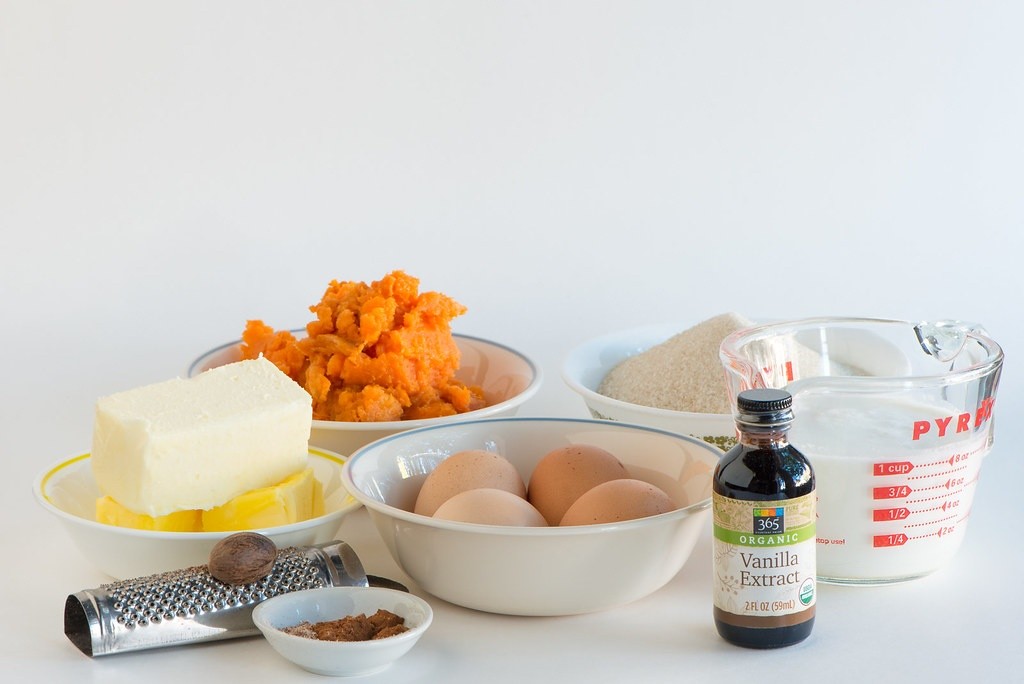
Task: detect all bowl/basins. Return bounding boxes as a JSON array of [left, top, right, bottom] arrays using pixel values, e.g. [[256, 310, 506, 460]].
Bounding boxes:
[[562, 317, 910, 454], [339, 416, 726, 617], [186, 326, 546, 459], [35, 445, 364, 582]]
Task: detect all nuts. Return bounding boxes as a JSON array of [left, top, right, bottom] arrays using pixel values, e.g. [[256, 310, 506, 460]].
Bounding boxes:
[[206, 533, 280, 586]]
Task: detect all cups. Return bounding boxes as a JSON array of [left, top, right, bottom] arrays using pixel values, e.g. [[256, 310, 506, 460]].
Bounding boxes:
[[718, 318, 1004, 587]]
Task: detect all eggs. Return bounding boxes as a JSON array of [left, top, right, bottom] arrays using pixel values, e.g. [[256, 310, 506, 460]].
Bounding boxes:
[[410, 443, 678, 527]]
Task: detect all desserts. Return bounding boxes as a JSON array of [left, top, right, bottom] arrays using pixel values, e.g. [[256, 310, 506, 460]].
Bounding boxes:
[[82, 354, 326, 532]]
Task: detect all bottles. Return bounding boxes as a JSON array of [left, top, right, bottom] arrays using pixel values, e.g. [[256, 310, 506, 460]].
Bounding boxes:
[[711, 389, 818, 650]]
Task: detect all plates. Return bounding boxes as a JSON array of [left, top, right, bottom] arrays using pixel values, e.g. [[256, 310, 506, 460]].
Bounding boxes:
[[253, 585, 433, 679]]
